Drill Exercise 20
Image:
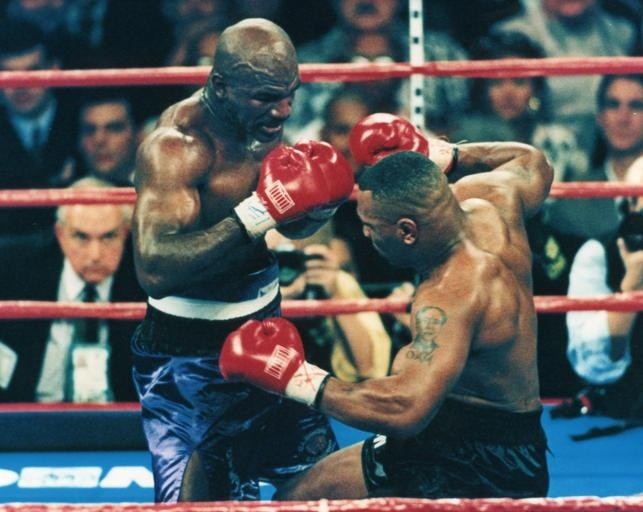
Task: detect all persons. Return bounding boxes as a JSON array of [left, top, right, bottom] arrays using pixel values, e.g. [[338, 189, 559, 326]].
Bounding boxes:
[[125, 13, 357, 506], [211, 110, 558, 506], [0, 0, 643, 414]]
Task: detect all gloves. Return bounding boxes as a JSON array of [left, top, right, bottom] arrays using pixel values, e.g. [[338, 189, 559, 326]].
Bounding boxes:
[[233, 146, 330, 250], [293, 138, 356, 223], [346, 113, 459, 179], [214, 317, 331, 409]]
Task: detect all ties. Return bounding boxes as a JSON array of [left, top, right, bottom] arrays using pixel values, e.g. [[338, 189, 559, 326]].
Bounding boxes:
[[60, 284, 104, 402]]
